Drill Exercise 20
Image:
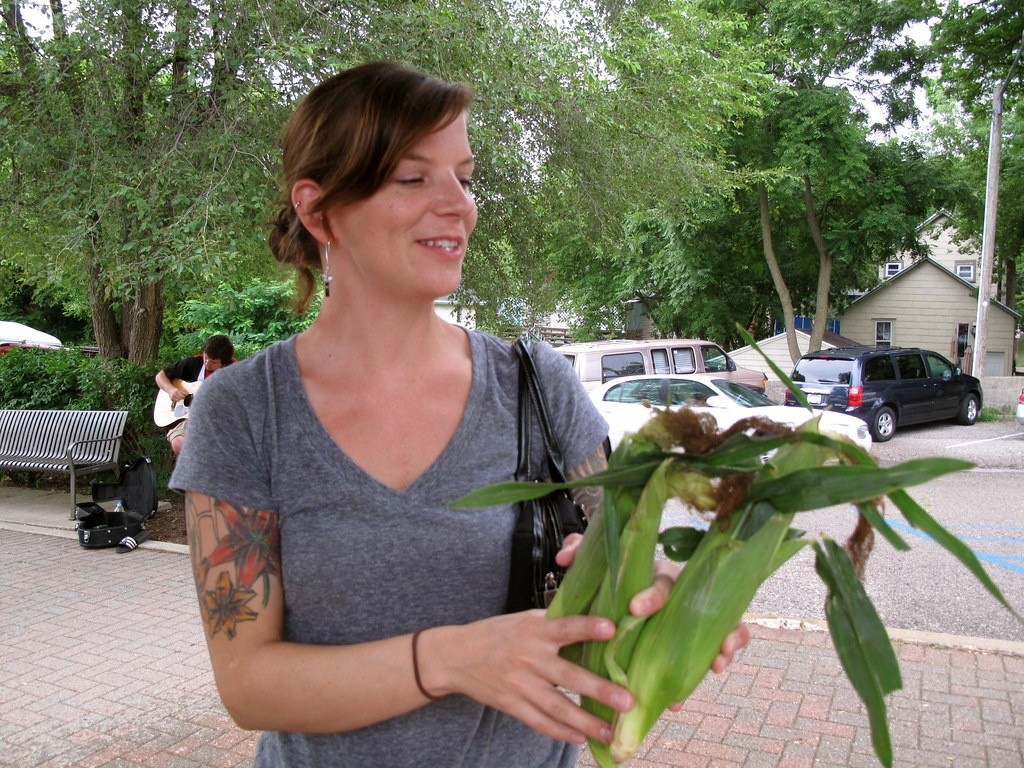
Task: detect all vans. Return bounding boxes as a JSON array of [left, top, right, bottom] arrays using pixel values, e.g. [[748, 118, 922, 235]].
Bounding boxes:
[[556, 339, 767, 420]]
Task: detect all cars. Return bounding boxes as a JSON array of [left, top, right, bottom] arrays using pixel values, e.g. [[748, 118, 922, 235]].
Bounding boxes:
[[588, 371, 872, 467]]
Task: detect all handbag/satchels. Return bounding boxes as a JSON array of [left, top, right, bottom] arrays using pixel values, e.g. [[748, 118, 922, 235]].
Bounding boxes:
[[505, 332, 591, 690]]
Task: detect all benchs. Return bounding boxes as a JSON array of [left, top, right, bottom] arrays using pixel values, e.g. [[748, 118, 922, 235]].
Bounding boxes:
[[0, 409, 130, 521]]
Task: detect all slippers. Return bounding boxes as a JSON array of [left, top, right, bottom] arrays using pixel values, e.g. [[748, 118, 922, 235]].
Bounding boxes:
[[116, 530, 150, 554]]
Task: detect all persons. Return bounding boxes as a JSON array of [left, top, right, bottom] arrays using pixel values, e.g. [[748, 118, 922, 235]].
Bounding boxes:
[[688, 392, 709, 407], [155, 334, 240, 456], [167, 59, 749, 768]]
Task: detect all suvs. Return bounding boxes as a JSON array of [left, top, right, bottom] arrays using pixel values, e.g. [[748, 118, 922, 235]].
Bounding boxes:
[[787, 343, 983, 443]]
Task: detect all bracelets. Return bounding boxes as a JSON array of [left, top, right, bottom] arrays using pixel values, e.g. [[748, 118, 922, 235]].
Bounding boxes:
[[411, 626, 444, 701]]
